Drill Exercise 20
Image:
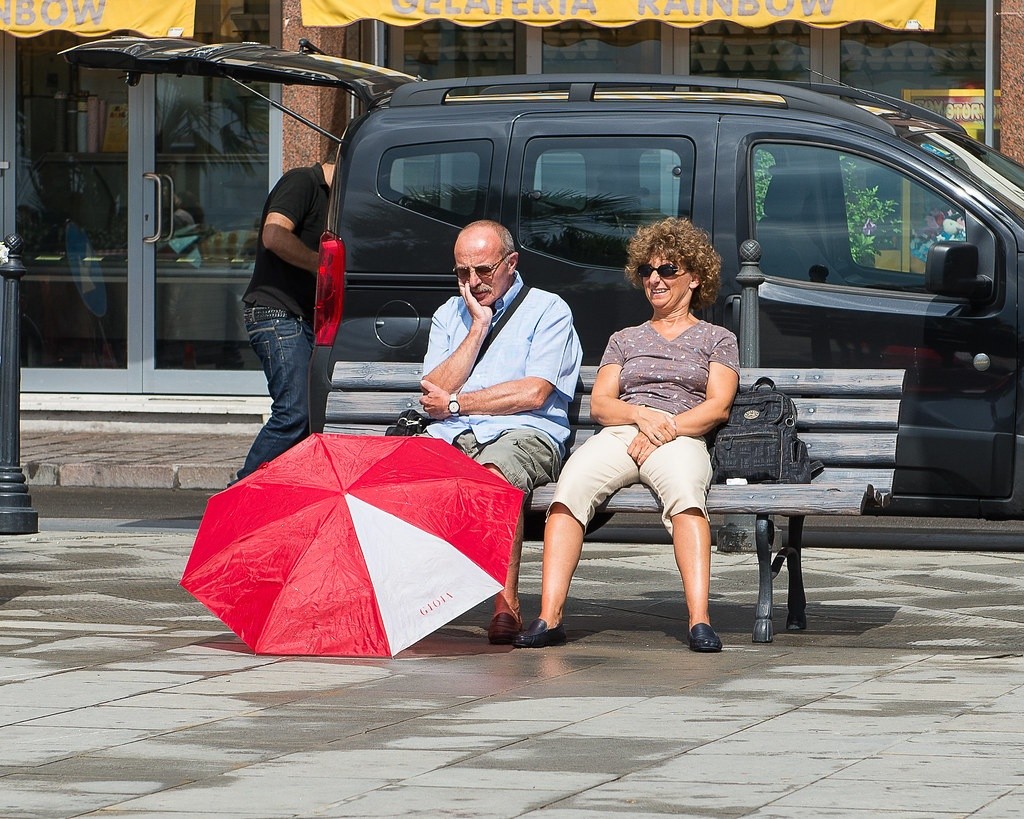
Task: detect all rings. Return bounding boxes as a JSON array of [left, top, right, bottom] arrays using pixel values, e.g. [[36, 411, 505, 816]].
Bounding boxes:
[[641, 452, 643, 455], [657, 434, 662, 438]]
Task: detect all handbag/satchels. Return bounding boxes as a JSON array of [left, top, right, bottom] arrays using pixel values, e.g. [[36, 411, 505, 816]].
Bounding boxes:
[[384, 409, 430, 436]]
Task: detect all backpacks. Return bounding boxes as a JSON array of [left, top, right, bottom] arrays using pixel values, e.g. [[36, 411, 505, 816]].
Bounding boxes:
[[709, 377, 826, 484]]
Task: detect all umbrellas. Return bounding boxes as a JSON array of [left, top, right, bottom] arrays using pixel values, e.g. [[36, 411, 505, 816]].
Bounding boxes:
[[181, 434, 526, 657]]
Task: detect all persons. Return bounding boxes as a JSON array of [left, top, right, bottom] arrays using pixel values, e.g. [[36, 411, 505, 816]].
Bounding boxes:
[[510, 217, 740, 652], [225, 147, 338, 487], [414, 220, 584, 643]]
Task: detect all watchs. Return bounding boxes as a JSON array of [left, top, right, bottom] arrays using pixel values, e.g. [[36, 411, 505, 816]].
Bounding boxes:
[[449, 394, 460, 416]]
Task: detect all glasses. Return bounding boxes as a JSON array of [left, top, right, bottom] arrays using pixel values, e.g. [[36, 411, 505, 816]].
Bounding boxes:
[[453, 252, 512, 280], [636, 263, 690, 278]]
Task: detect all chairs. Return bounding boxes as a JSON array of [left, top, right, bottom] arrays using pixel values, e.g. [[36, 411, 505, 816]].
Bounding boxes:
[[755, 165, 847, 287]]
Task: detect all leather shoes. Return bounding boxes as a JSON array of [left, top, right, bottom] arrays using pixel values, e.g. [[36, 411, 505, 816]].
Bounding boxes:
[[512, 618, 567, 647], [488, 611, 522, 642], [687, 622, 723, 651]]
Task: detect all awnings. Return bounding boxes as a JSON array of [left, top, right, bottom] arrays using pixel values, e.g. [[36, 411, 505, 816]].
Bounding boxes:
[[299, 1, 938, 33], [0, 0, 196, 38]]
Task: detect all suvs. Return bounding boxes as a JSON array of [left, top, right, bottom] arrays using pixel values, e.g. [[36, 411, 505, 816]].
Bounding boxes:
[[57, 39, 1024, 522]]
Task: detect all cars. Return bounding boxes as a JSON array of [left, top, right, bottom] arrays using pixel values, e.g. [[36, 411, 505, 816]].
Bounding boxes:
[[17, 152, 268, 368]]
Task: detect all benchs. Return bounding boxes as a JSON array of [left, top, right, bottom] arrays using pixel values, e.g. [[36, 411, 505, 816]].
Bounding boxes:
[[321, 360, 908, 643]]
